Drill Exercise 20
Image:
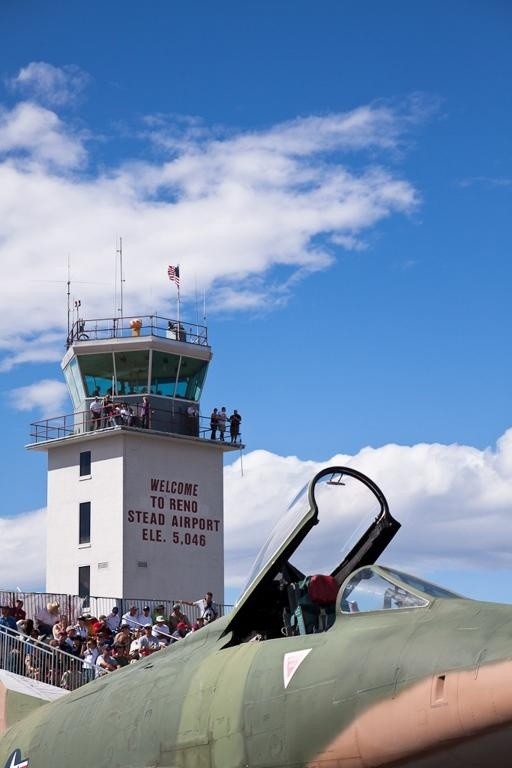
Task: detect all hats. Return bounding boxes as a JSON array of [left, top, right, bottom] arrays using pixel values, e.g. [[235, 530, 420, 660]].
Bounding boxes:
[[66, 604, 199, 650]]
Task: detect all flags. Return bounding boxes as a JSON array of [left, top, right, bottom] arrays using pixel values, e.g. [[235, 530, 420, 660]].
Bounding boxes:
[[168, 265, 179, 286]]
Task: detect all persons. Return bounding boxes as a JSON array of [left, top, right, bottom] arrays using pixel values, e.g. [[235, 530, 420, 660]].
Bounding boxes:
[[217, 407, 230, 441], [187, 402, 199, 437], [90, 383, 162, 430], [230, 410, 241, 443], [1, 591, 218, 691], [211, 408, 220, 440]]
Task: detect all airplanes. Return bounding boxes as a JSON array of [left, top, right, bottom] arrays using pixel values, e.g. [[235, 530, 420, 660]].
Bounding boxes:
[[0, 462, 512, 768]]
